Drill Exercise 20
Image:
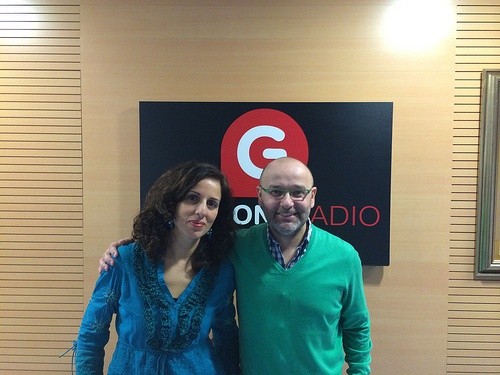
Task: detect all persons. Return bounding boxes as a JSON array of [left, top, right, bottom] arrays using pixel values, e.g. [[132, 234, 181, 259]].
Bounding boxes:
[[96, 157, 373, 375], [75, 162, 240, 375]]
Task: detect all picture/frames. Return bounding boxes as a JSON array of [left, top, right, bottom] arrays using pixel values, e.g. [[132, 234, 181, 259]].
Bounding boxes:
[[474, 68, 500, 279]]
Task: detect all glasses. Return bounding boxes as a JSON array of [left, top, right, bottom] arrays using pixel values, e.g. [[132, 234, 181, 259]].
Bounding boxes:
[[260, 186, 313, 201]]
